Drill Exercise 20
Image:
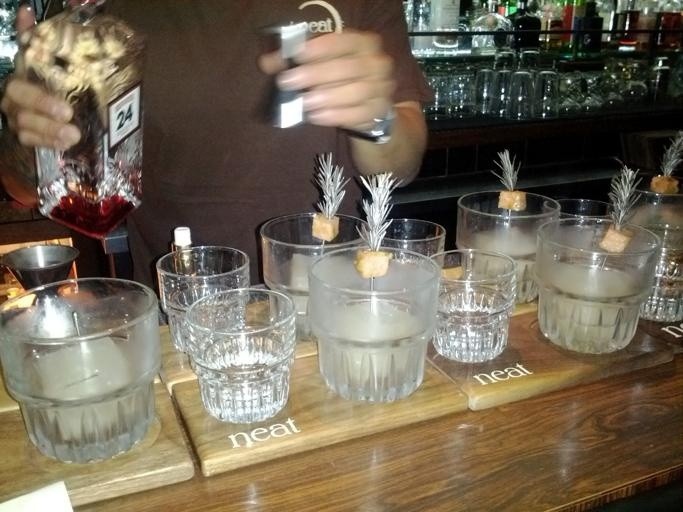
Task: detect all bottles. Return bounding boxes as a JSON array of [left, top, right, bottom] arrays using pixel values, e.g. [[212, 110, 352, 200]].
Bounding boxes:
[[14, 0, 144, 241], [176, 228, 200, 296], [467, 0, 682, 52]]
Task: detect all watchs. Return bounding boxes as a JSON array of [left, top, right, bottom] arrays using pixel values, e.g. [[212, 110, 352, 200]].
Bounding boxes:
[[347, 103, 395, 145]]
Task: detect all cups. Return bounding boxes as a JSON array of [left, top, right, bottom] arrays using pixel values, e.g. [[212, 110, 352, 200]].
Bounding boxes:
[[630, 220, 683, 321], [157, 245, 251, 350], [536, 219, 661, 356], [612, 168, 683, 223], [543, 199, 616, 217], [0, 277, 161, 467], [308, 247, 440, 403], [429, 249, 513, 364], [261, 215, 367, 342], [379, 218, 445, 261], [3, 244, 78, 296], [424, 53, 670, 118], [457, 190, 561, 304], [185, 288, 296, 425]]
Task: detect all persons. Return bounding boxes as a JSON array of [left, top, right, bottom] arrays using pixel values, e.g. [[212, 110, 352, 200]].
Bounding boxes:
[[0, 0, 435, 327]]
